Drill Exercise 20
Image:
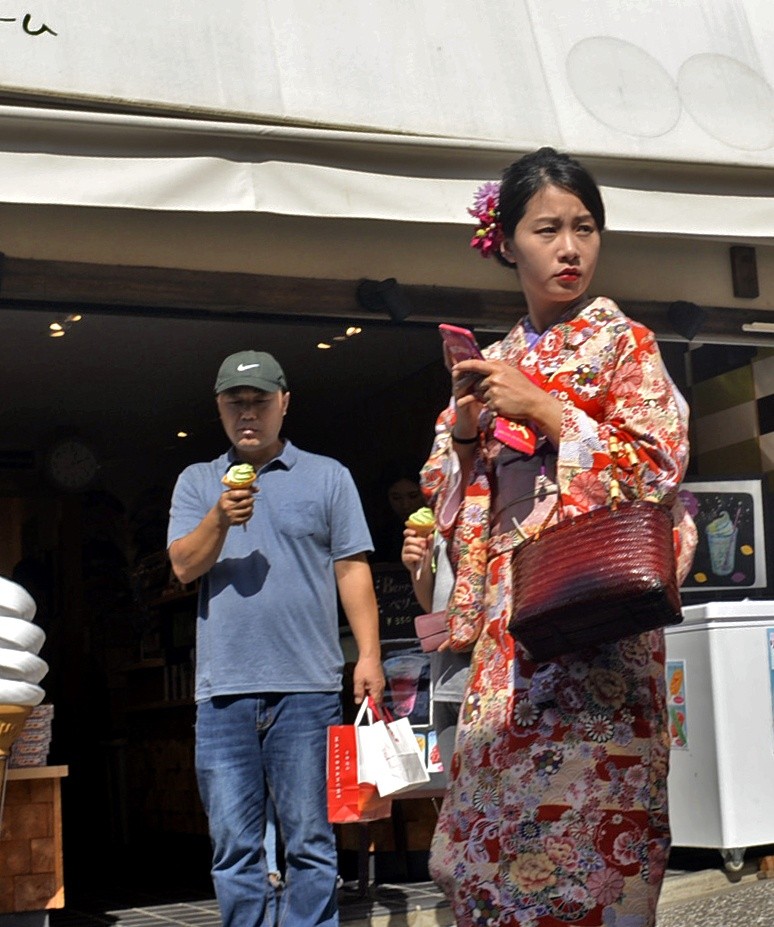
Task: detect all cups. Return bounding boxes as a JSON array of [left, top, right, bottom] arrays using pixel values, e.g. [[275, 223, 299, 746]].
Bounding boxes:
[[708, 528, 738, 576], [383, 656, 425, 716]]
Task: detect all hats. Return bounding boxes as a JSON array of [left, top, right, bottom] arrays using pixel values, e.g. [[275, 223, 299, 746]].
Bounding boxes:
[[214, 350, 286, 392]]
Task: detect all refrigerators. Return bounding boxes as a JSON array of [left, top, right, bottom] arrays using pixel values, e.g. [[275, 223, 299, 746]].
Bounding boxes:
[[662, 599, 774, 872]]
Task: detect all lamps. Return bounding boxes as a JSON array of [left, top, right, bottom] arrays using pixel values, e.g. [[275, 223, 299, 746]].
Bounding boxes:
[[669, 301, 706, 340], [359, 277, 416, 323]]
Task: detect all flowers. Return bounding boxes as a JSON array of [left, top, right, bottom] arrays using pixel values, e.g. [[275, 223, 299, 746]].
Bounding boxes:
[[467, 181, 504, 257]]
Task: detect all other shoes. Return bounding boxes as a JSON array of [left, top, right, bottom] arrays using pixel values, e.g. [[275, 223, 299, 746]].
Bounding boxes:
[[268, 874, 285, 891]]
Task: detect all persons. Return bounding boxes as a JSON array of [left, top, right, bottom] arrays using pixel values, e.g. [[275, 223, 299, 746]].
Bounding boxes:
[[402, 506, 472, 782], [388, 471, 421, 524], [420, 147, 699, 927], [165, 348, 387, 927]]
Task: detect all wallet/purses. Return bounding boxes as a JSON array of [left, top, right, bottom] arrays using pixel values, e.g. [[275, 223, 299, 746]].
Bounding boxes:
[[414, 611, 450, 653]]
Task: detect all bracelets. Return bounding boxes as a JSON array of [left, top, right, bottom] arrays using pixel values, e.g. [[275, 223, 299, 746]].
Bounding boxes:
[[449, 427, 480, 446]]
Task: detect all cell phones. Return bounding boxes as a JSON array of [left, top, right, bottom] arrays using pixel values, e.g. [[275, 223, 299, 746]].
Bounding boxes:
[[437, 321, 486, 368]]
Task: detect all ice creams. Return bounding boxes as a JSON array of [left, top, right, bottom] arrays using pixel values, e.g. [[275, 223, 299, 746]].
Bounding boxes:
[[405, 507, 437, 581], [0, 577, 48, 800], [221, 463, 257, 531]]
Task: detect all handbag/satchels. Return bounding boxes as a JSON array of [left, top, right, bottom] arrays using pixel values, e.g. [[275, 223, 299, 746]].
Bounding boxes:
[[355, 696, 431, 798], [507, 432, 684, 662], [326, 696, 392, 823]]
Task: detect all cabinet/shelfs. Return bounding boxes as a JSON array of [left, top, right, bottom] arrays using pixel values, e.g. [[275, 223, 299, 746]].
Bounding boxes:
[[108, 544, 203, 850]]
